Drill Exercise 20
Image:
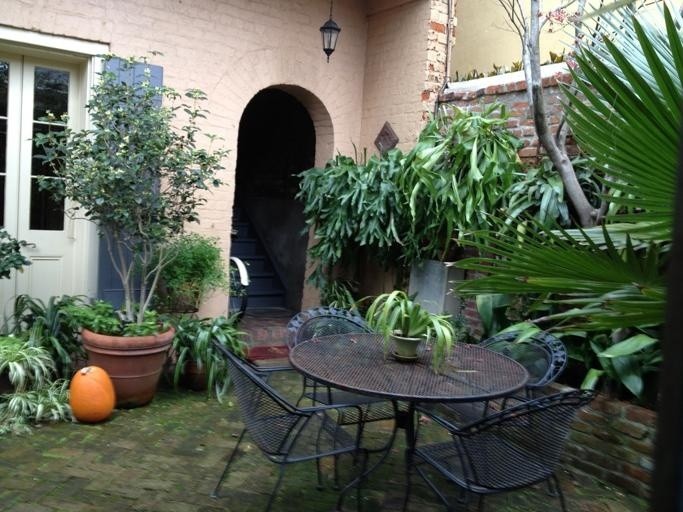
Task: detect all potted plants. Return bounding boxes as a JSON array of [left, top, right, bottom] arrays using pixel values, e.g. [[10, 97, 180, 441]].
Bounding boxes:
[[364, 290, 456, 376]]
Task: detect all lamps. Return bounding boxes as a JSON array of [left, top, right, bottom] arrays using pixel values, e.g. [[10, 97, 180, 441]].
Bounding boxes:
[[320, 0, 341, 63]]
